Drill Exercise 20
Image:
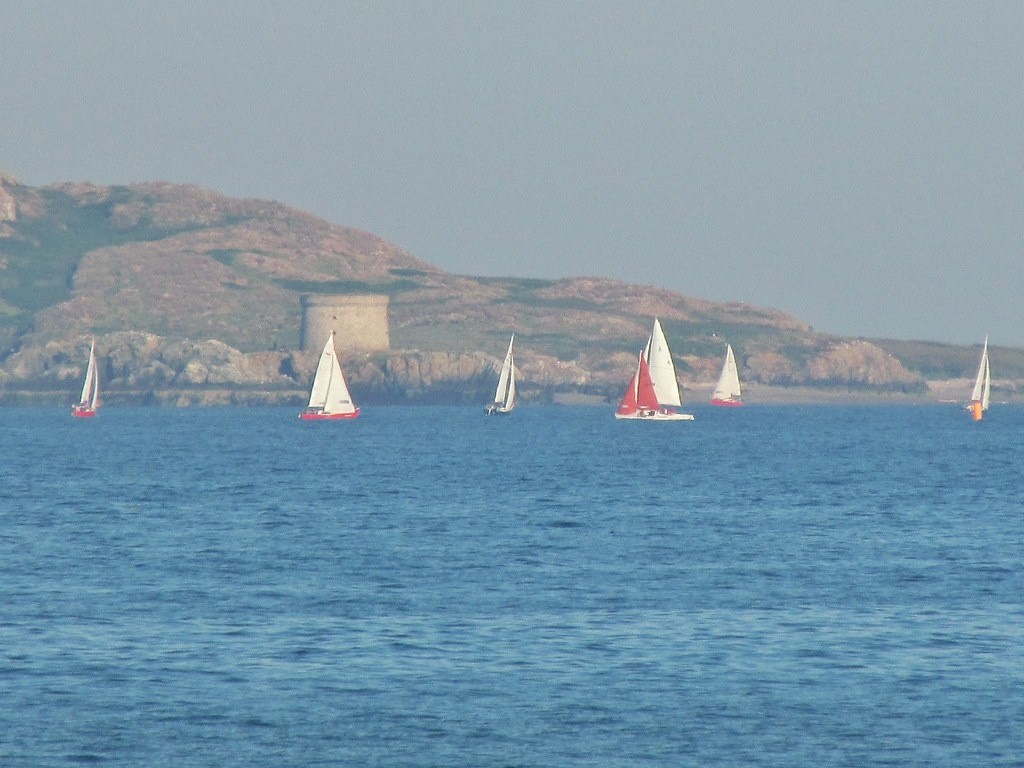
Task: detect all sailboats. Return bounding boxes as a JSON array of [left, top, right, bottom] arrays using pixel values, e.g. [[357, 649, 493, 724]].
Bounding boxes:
[[299, 330, 360, 420], [483, 332, 516, 415], [711, 344, 743, 406], [70, 341, 99, 417], [615, 318, 694, 421], [963, 337, 991, 412]]
